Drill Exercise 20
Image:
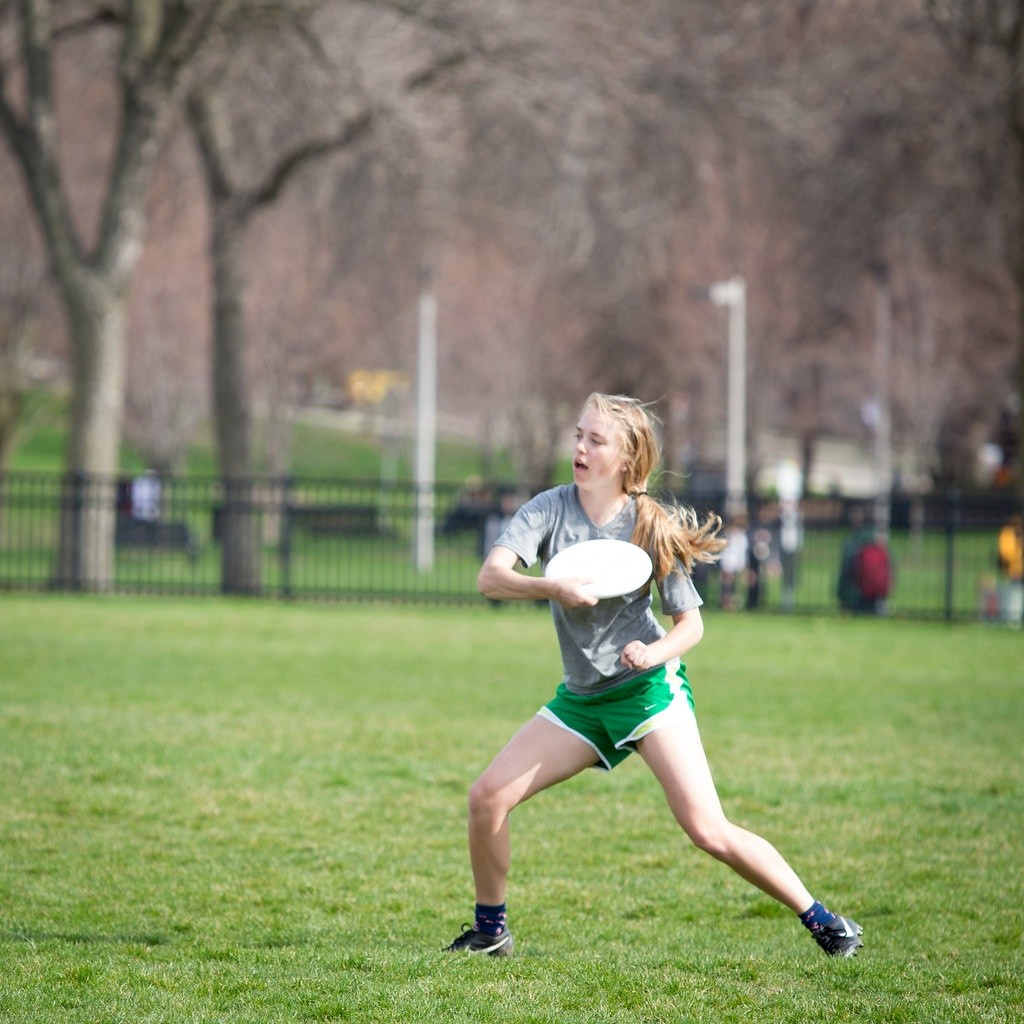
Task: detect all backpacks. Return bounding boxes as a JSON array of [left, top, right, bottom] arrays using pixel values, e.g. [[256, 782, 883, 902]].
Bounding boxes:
[[857, 541, 890, 598]]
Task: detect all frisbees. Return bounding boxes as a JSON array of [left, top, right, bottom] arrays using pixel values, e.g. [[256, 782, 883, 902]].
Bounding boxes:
[[545, 539, 653, 599]]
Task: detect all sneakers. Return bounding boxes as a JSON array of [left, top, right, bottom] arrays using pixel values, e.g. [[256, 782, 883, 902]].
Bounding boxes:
[[442, 922, 513, 957], [811, 913, 864, 961]]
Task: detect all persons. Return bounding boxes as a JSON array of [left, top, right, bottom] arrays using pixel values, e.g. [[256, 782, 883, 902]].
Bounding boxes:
[[433, 390, 865, 964], [740, 497, 1024, 630]]
